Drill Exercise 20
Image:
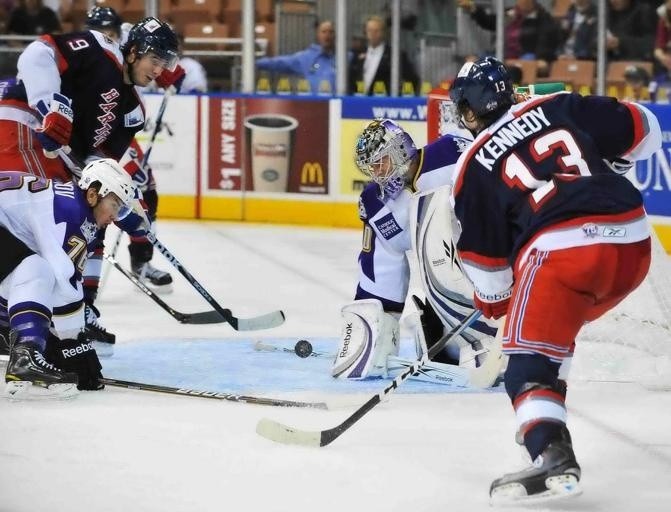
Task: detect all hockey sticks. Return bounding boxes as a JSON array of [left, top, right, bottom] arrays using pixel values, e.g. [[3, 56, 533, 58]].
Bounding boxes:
[[102, 253, 232, 324], [257, 307, 482, 446], [254, 317, 506, 389], [58, 149, 285, 332]]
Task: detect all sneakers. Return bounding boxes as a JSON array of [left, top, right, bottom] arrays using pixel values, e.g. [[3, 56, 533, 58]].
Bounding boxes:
[[86, 307, 117, 344], [131, 258, 173, 286], [0, 328, 14, 354], [488, 429, 581, 500], [6, 345, 78, 385]]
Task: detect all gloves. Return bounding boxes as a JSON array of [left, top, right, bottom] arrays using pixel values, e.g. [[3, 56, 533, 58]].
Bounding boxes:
[[472, 288, 515, 320], [120, 147, 149, 187], [34, 94, 76, 149], [78, 372, 107, 392]]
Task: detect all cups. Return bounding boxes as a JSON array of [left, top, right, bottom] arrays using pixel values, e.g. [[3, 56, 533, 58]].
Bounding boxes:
[[242, 114, 300, 195]]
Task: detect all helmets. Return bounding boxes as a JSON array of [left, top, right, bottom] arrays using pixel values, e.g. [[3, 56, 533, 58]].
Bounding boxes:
[[357, 117, 418, 199], [86, 5, 122, 40], [125, 16, 179, 74], [450, 57, 513, 136], [623, 66, 650, 86], [76, 158, 135, 221]]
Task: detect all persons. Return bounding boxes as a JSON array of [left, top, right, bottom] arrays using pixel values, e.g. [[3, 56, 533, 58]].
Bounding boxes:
[[463, 1, 671, 104], [333, 117, 506, 390], [258, 12, 419, 98], [452, 57, 663, 500], [1, 0, 206, 402]]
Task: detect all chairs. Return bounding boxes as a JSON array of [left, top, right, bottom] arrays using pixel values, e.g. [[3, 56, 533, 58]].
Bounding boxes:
[[504, 59, 655, 99], [57, 0, 312, 92]]
